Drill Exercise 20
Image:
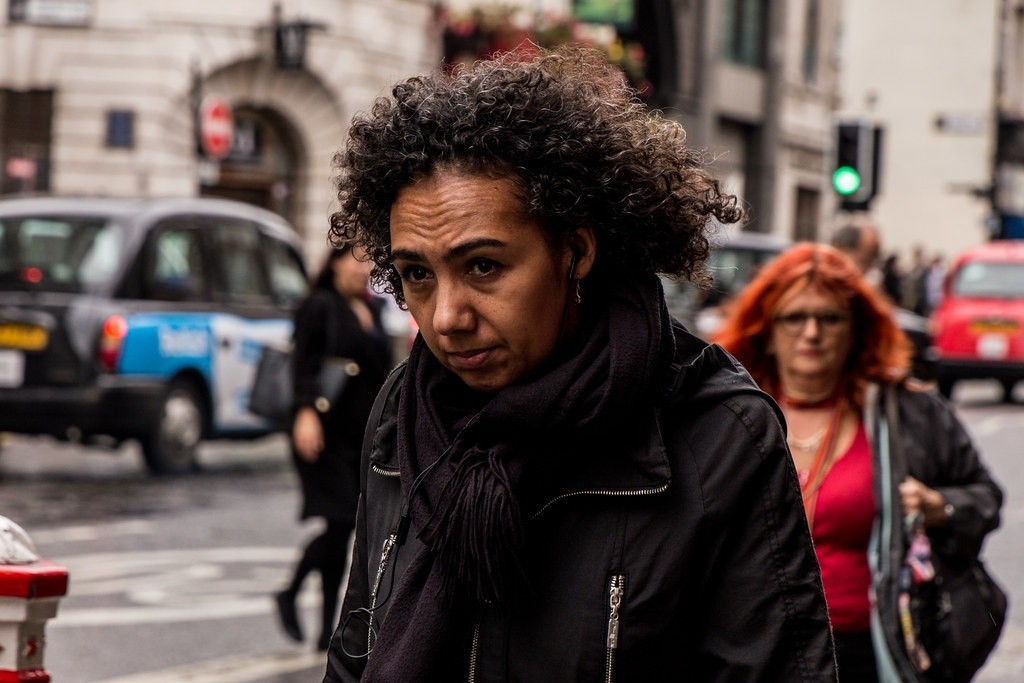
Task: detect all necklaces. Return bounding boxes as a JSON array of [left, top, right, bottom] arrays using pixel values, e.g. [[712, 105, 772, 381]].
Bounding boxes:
[[778, 424, 829, 451]]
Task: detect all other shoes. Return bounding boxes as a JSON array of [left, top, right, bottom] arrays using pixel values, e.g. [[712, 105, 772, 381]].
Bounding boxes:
[[276, 591, 301, 641], [316, 624, 334, 650]]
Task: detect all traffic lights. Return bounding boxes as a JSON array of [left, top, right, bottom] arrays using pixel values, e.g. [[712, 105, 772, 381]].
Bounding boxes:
[[833, 120, 882, 206]]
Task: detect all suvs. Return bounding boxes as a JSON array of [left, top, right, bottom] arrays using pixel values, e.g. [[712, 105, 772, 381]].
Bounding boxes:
[[0, 190, 315, 477]]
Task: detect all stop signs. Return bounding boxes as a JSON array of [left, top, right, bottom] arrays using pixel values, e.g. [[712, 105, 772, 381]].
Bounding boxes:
[[200, 94, 235, 158]]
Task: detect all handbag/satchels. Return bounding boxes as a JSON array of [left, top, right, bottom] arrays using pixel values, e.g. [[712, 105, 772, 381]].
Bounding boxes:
[[885, 381, 1007, 683], [247, 290, 360, 428]]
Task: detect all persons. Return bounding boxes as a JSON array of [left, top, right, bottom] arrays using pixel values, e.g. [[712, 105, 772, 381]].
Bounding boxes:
[[687, 218, 1003, 683], [840, 226, 955, 320], [270, 240, 396, 655], [321, 46, 837, 683]]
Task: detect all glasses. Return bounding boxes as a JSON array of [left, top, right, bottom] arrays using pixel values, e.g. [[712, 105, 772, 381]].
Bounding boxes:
[[770, 307, 858, 336]]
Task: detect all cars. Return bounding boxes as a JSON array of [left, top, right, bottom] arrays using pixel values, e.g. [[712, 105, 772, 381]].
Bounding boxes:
[[927, 239, 1024, 403], [659, 229, 938, 386]]
